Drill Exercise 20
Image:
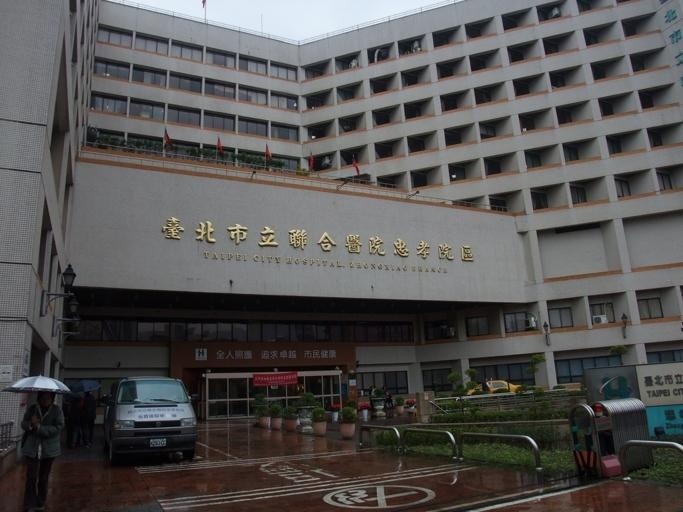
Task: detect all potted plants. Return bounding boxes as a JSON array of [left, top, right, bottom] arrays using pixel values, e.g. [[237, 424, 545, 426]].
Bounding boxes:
[[251, 392, 329, 437], [337, 406, 359, 439], [370, 387, 387, 409], [394, 395, 404, 416]]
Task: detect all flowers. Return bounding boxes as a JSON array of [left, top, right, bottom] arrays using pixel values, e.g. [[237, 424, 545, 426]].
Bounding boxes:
[[406, 399, 416, 405], [357, 401, 370, 409], [329, 402, 342, 411]]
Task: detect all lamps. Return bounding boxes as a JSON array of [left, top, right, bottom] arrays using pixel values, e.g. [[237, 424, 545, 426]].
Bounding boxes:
[[621, 312, 628, 337], [543, 321, 549, 344], [40, 263, 80, 337]]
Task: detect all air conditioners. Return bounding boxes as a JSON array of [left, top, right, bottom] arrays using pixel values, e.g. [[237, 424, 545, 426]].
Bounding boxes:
[[592, 315, 607, 325]]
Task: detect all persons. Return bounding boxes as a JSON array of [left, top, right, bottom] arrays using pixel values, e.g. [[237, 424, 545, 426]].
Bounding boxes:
[[86, 392, 97, 449], [20, 392, 65, 512], [63, 396, 85, 447]]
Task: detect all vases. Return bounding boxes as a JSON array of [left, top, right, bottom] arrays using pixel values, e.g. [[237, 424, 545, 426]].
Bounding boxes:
[[408, 406, 415, 414], [362, 408, 369, 420], [331, 411, 339, 422]]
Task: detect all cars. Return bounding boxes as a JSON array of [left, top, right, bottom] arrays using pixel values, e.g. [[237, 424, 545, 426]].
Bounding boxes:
[[463, 378, 525, 395]]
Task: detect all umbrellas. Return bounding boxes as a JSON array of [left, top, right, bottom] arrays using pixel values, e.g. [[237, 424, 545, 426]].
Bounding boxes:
[[2, 373, 72, 396], [63, 377, 104, 394]]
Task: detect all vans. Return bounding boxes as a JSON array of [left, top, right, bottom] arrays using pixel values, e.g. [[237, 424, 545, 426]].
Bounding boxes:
[[100, 376, 200, 466]]
